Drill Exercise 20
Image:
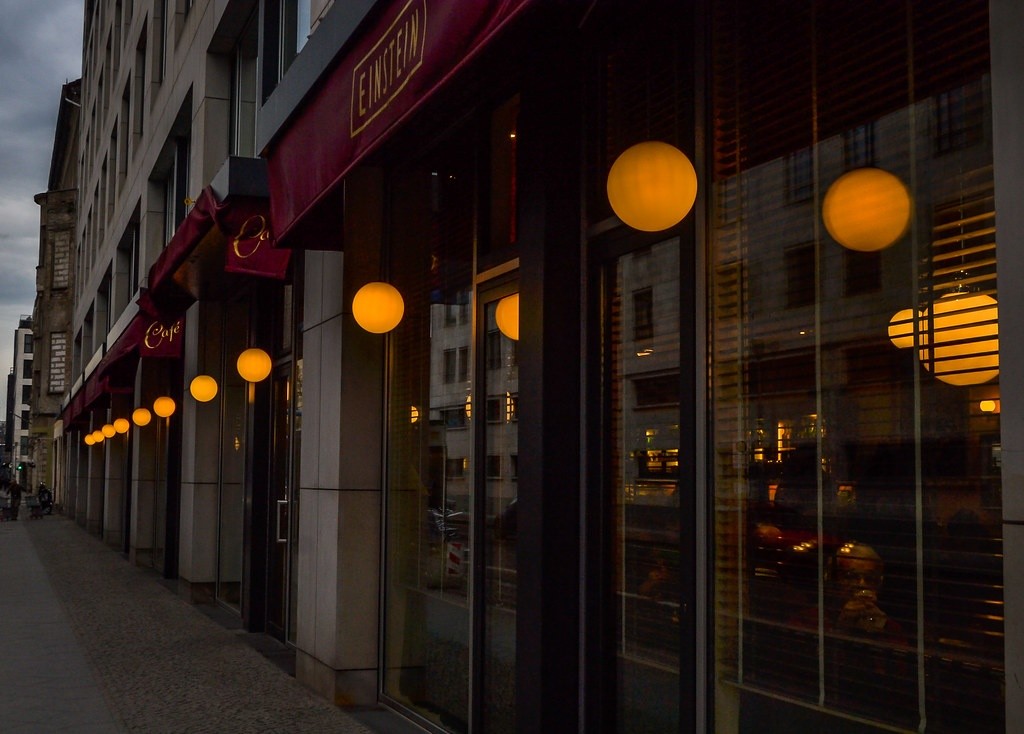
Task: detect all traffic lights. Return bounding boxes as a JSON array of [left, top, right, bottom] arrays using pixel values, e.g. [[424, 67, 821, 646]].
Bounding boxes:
[[17, 464, 22, 471]]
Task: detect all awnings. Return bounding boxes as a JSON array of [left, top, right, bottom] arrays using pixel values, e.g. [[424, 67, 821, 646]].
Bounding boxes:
[[148, 186, 291, 317], [269, 0, 537, 249], [62, 287, 182, 432]]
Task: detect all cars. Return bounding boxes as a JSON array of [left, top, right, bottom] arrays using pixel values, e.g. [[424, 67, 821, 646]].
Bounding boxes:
[[496, 487, 836, 612]]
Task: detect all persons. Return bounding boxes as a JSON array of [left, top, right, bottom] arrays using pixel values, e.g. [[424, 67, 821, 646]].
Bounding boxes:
[[638, 512, 682, 646], [6, 480, 27, 507]]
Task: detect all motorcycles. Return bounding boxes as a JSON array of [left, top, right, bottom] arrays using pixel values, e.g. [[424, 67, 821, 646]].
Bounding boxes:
[[38, 482, 53, 515]]
